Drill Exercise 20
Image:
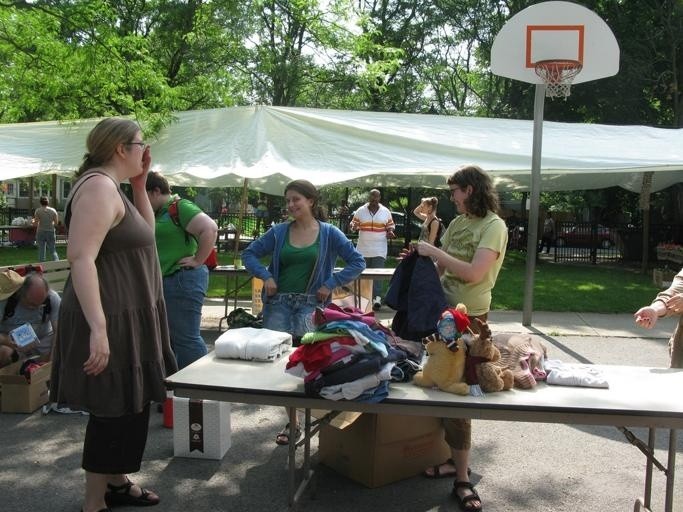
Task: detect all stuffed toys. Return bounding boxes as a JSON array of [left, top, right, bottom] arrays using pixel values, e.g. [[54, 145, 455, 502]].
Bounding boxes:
[[462, 318, 515, 392], [411, 337, 471, 395]]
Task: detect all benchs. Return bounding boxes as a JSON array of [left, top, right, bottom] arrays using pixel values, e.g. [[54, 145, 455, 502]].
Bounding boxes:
[[0, 260, 72, 317]]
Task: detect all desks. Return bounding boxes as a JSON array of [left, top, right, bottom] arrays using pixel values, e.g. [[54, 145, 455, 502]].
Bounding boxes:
[[213, 265, 396, 333], [164, 347, 683, 512], [0, 225, 37, 249]]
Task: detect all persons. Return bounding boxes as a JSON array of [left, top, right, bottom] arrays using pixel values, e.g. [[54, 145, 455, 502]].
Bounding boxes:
[[146, 170, 220, 413], [0, 272, 62, 367], [51, 117, 179, 512], [220, 196, 229, 222], [538, 210, 555, 255], [634, 265, 683, 369], [268, 194, 281, 229], [280, 208, 289, 226], [349, 190, 396, 310], [394, 166, 510, 512], [336, 198, 349, 234], [254, 190, 270, 237], [240, 181, 366, 446], [413, 193, 445, 247], [33, 195, 59, 264]]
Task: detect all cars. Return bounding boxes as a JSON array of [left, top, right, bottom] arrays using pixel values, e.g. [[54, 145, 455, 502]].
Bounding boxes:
[[556, 222, 614, 249], [390, 212, 422, 238]]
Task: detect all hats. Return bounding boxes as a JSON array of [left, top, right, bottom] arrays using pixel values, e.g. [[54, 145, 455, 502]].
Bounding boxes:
[[0, 268, 26, 301]]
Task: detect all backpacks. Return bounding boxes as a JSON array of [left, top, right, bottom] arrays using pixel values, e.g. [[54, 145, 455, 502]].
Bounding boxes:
[[426, 216, 447, 247]]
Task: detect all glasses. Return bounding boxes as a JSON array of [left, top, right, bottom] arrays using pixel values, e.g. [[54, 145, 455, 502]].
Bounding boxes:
[[124, 141, 146, 151], [450, 188, 462, 195]]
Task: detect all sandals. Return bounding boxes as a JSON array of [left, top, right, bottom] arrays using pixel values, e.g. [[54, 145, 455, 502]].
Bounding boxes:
[[423, 458, 470, 478], [451, 480, 482, 512], [105, 476, 160, 505], [277, 422, 301, 444]]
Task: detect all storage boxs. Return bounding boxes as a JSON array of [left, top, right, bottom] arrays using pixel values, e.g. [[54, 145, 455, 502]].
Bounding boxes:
[[173, 396, 231, 460], [296, 408, 452, 488], [0, 355, 52, 413]]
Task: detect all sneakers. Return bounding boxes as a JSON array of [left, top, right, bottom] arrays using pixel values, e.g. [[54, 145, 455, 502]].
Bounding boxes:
[[371, 295, 382, 312]]
